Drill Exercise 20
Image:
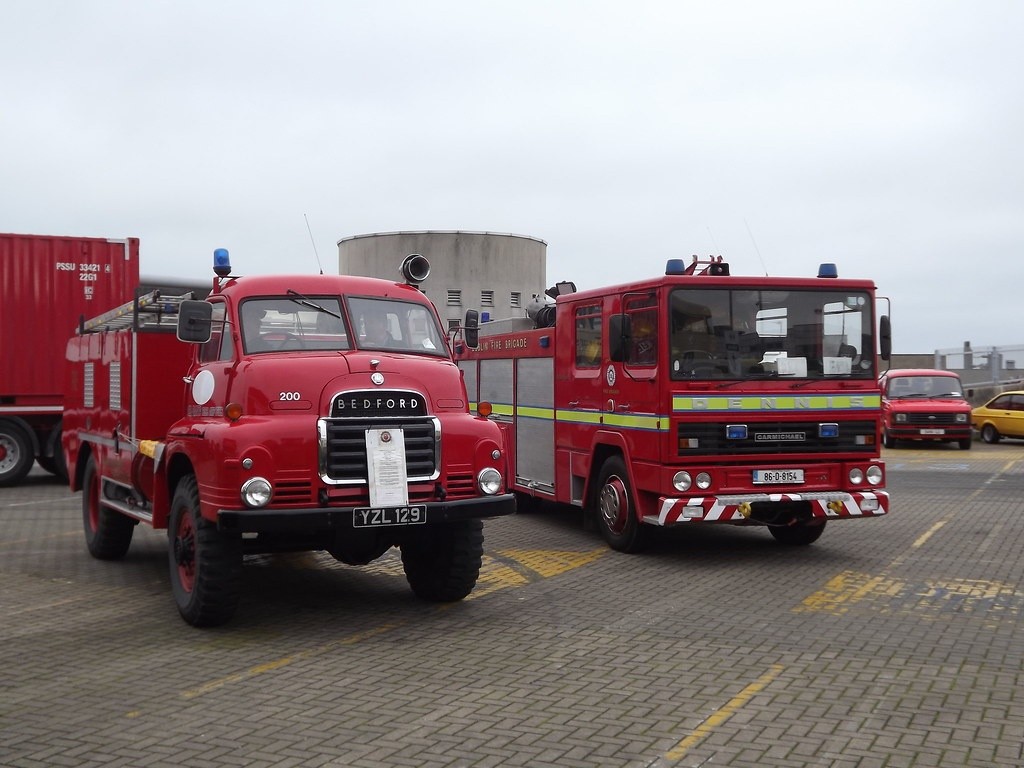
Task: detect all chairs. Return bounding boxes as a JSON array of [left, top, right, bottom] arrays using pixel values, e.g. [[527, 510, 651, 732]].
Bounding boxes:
[[676, 332, 712, 378], [895, 380, 913, 395]]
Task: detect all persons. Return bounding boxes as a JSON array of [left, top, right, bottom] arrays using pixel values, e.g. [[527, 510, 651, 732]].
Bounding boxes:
[[363, 308, 407, 348], [241, 313, 281, 356]]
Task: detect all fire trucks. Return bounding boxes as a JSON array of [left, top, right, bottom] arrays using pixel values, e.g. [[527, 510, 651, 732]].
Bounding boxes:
[[445, 253, 892, 552], [62, 247, 517, 630]]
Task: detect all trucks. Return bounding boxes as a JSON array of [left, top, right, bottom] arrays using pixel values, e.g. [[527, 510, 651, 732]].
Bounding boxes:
[[877, 366, 973, 453]]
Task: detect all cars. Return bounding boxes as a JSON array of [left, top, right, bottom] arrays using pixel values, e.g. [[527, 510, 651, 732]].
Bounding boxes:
[[970, 390, 1024, 444]]
